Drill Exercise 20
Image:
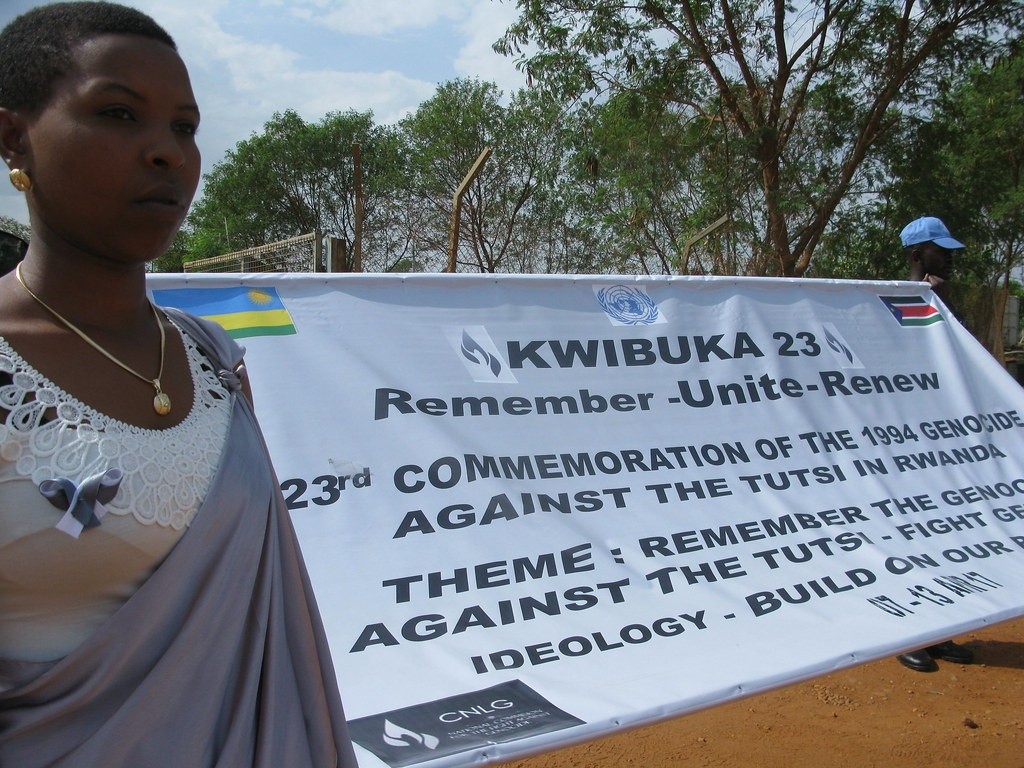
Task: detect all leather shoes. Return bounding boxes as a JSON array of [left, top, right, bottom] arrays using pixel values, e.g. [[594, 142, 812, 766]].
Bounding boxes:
[[896, 648, 932, 671], [926, 640, 974, 664]]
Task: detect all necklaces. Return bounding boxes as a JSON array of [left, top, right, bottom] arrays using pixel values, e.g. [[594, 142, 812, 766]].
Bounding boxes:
[[16, 258, 172, 415]]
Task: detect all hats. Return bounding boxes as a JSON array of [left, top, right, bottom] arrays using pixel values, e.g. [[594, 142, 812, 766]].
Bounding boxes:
[[899, 216, 966, 249]]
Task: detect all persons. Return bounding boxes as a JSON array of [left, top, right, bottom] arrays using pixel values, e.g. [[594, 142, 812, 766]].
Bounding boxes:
[[0, 0, 360, 768], [896, 216, 974, 672]]
